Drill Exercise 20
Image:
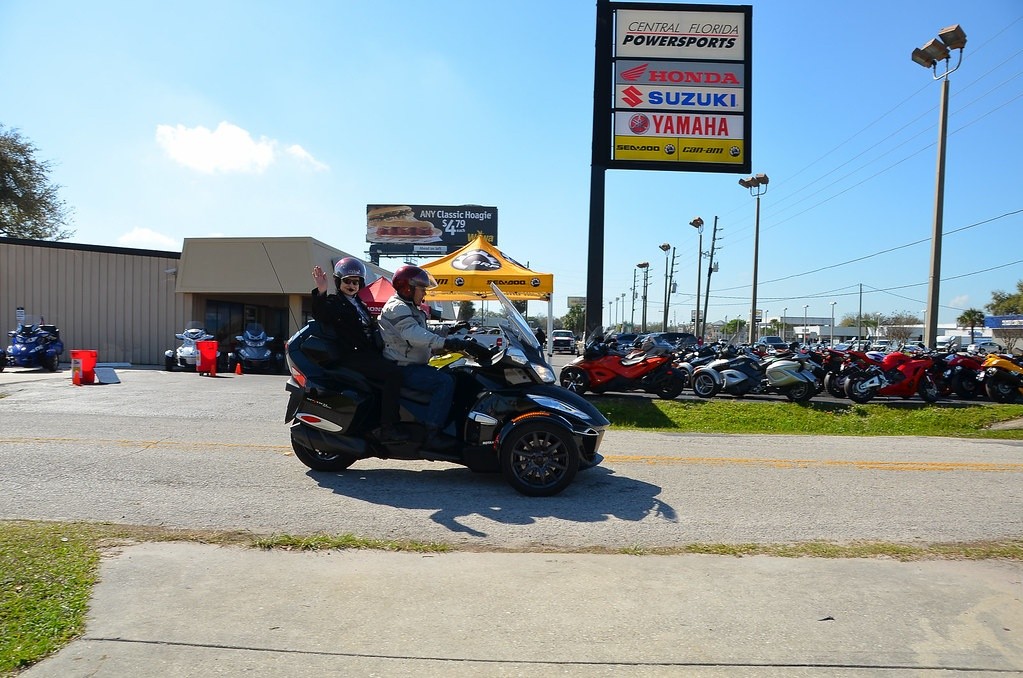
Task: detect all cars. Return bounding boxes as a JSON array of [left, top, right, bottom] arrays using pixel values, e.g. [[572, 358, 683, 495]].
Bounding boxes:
[[758, 336, 788, 350], [553, 329, 575, 355], [632, 333, 713, 356], [614, 332, 641, 351]]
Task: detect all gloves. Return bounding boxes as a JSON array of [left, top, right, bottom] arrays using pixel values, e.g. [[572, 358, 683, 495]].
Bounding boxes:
[[444, 338, 461, 350], [448, 320, 471, 335]]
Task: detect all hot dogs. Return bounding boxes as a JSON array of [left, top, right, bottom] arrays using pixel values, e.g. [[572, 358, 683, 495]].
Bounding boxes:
[[367, 205, 434, 238]]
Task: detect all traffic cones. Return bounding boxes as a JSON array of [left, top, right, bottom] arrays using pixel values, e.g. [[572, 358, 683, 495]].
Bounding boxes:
[[234, 363, 243, 375]]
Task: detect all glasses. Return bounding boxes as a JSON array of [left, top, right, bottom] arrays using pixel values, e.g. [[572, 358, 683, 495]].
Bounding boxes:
[[343, 278, 360, 285]]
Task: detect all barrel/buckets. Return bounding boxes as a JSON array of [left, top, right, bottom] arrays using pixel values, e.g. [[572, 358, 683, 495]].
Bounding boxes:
[[69, 349, 99, 385], [195, 340, 218, 372]]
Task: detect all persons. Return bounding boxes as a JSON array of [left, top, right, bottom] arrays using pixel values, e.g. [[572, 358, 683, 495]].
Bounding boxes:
[[310, 256, 412, 445], [534, 327, 546, 363], [376, 256, 495, 452]]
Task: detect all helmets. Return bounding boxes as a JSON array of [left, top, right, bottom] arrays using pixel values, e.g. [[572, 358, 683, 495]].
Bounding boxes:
[[332, 257, 366, 290], [393, 266, 429, 301]]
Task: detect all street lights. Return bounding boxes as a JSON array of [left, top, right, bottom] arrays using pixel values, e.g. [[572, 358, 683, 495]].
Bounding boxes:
[[830, 301, 837, 348], [635, 262, 650, 331], [803, 305, 810, 345], [690, 217, 704, 335], [738, 173, 769, 346], [783, 307, 788, 340], [737, 314, 741, 332], [659, 242, 670, 330], [911, 23, 966, 348], [764, 309, 769, 335]]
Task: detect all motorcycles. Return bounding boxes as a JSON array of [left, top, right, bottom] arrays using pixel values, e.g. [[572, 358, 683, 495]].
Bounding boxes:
[[164, 321, 221, 374], [0, 324, 63, 373], [227, 324, 285, 374], [285, 282, 612, 496], [559, 324, 1023, 407]]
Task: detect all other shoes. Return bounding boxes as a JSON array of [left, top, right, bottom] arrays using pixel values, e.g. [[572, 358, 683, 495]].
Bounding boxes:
[[380, 423, 412, 444], [422, 429, 458, 453]]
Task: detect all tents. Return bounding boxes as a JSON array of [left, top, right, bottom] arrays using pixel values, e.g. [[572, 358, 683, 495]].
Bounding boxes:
[[413, 231, 555, 368], [355, 275, 431, 326]]
